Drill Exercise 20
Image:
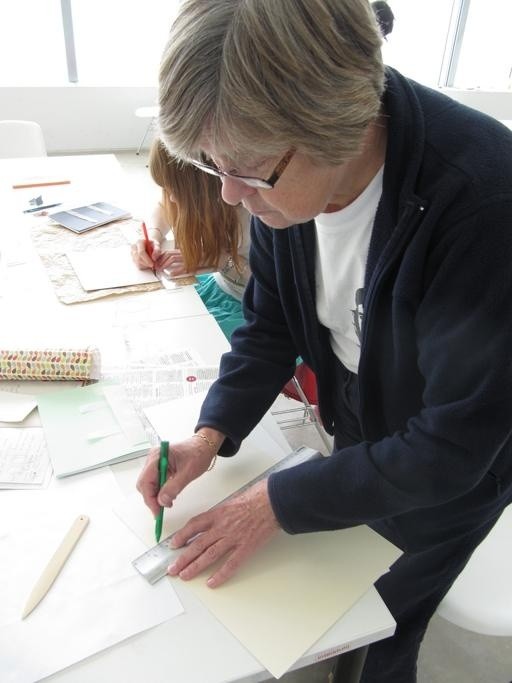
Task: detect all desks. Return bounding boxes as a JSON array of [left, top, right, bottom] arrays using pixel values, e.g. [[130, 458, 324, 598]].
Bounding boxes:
[[0, 154, 397, 680]]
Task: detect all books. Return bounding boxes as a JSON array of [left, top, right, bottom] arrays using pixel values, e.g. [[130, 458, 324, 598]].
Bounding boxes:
[[48, 202, 130, 234], [35, 380, 165, 479]]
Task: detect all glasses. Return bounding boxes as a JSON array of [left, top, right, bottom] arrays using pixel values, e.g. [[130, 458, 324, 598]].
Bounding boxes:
[[191, 147, 297, 191]]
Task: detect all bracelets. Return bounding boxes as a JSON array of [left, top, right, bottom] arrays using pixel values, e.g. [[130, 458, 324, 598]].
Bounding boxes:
[[148, 227, 163, 237], [192, 433, 217, 471]]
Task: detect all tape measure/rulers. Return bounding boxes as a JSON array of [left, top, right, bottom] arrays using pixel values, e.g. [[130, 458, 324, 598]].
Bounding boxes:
[[121, 229, 179, 290], [131, 445, 325, 584]]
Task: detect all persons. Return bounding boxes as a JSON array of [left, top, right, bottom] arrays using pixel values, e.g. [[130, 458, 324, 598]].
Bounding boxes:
[[131, 136, 253, 346], [136, 0, 511, 683]]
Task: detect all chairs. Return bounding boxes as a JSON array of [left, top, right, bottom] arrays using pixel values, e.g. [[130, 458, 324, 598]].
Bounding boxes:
[[135, 104, 162, 155], [0, 121, 49, 156]]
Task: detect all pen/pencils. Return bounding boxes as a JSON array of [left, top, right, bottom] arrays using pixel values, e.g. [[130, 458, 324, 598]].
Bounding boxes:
[[155, 441, 169, 542], [24, 203, 62, 213], [13, 181, 72, 188], [142, 222, 157, 276]]
